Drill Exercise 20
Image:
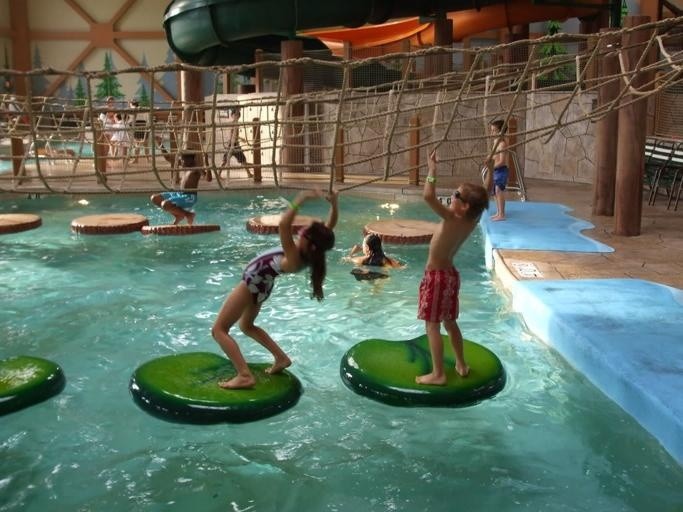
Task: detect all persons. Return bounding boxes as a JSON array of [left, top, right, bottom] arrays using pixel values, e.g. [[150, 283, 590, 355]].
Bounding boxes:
[[1, 78, 23, 132], [217, 111, 254, 179], [99, 95, 151, 164], [149, 134, 201, 225], [415, 147, 494, 385], [486, 119, 510, 222], [341, 233, 408, 309], [212, 186, 340, 389]]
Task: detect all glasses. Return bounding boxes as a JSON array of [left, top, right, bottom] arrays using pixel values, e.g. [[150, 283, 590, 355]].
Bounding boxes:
[[455, 191, 465, 203], [297, 228, 315, 244]]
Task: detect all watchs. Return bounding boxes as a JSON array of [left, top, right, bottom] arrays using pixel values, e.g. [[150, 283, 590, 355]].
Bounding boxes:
[[425, 175, 437, 184]]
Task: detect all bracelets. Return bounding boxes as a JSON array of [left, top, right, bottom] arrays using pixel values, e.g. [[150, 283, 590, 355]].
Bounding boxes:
[[285, 196, 305, 217]]
